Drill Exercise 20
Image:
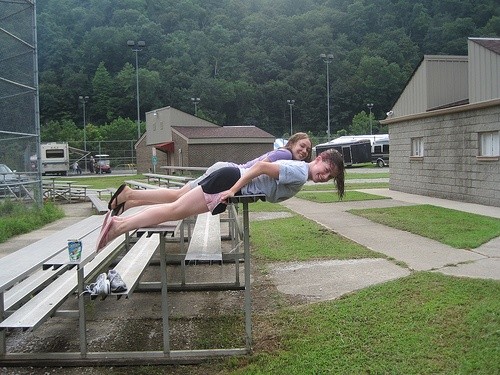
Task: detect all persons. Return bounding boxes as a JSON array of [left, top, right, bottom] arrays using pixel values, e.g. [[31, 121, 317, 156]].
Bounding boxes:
[[102, 133, 312, 215], [98, 148, 345, 252], [74, 161, 81, 175], [89, 156, 95, 173]]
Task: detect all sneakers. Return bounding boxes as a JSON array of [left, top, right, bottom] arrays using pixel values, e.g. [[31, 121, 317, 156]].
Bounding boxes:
[[107, 270, 127, 293], [79, 272, 111, 297]]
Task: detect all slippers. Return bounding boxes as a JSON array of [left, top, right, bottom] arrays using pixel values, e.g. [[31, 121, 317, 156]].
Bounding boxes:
[[99, 209, 114, 233], [108, 183, 127, 210], [111, 202, 126, 216], [95, 217, 115, 252]]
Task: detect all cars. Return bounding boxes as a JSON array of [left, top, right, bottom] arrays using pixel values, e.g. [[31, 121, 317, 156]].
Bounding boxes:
[[0, 164, 29, 196]]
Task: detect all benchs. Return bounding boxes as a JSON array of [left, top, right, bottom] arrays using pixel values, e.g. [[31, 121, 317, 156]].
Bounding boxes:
[[0, 165, 267, 366]]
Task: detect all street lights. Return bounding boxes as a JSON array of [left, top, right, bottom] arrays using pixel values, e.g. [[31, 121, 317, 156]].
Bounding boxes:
[[126, 40, 146, 139], [320, 53, 336, 141], [286, 99, 295, 136], [191, 97, 201, 117], [367, 103, 373, 135], [78, 95, 90, 171]]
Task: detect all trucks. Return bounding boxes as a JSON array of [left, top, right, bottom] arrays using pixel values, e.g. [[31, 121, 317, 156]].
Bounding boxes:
[[94, 154, 111, 174], [40, 141, 69, 176]]
